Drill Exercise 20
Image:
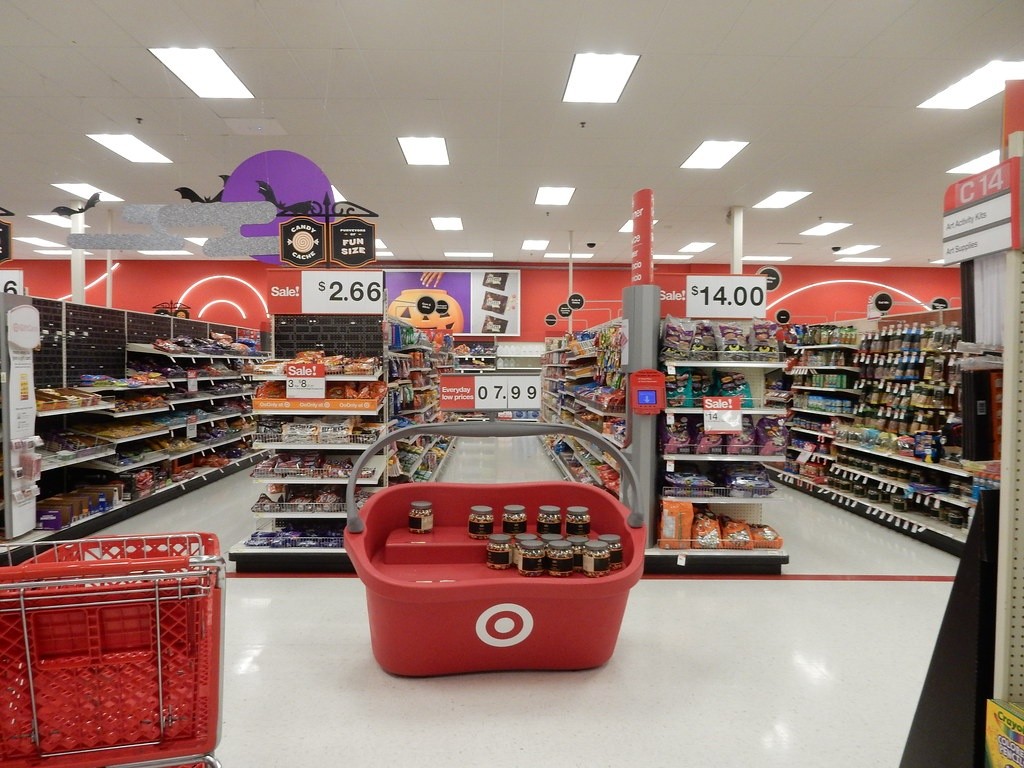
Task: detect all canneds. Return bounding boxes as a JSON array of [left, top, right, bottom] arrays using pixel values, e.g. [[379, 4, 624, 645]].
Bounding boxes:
[[826, 451, 973, 530], [408, 502, 624, 577]]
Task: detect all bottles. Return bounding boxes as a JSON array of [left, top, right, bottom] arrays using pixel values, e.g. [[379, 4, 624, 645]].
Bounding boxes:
[[789, 324, 859, 415], [407, 500, 434, 535], [466, 504, 624, 578]]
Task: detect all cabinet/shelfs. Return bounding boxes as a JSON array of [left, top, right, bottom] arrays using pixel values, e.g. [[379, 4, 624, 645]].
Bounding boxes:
[[388, 344, 457, 484], [2, 295, 268, 535], [644, 316, 789, 574], [538, 321, 626, 493], [762, 326, 1000, 559], [229, 363, 386, 579]]
